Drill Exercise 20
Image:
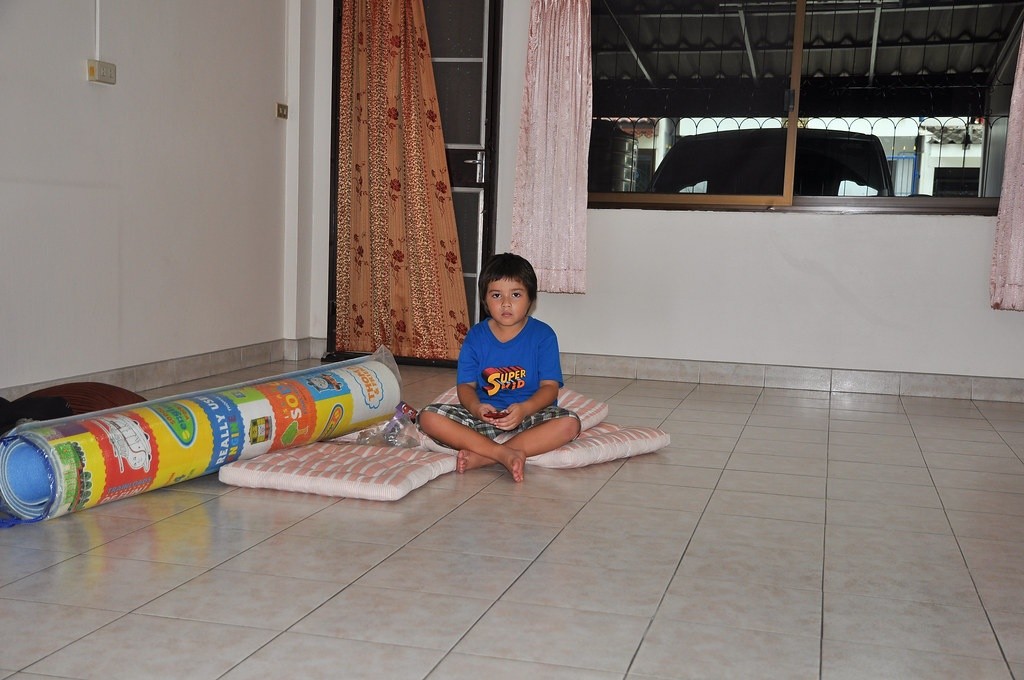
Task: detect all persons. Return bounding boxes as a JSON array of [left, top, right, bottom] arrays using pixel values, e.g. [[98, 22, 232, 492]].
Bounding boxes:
[[415, 252, 581, 482]]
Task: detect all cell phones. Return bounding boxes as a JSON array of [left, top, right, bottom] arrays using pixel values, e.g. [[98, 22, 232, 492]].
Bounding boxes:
[[484, 412, 508, 418]]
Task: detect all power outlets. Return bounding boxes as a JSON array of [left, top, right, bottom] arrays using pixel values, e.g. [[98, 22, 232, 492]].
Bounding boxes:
[[98, 60, 117, 84]]
